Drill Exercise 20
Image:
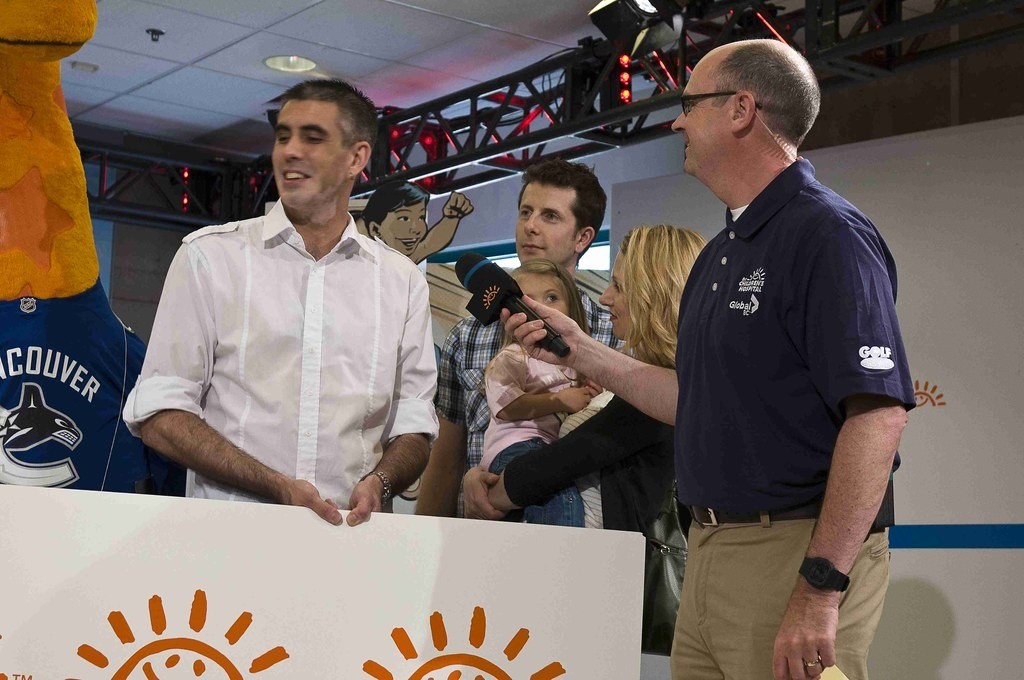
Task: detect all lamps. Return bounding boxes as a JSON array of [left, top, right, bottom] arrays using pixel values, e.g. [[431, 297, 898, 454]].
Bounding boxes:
[[589, 0, 683, 59]]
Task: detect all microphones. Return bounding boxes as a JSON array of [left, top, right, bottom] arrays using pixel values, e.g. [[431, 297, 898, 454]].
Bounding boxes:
[[455, 253, 570, 359]]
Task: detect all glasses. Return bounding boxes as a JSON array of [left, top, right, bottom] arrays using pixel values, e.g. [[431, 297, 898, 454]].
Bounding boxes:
[[680, 91, 763, 117]]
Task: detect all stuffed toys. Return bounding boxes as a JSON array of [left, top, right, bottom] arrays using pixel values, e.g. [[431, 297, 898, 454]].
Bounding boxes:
[[0, 0, 189, 499]]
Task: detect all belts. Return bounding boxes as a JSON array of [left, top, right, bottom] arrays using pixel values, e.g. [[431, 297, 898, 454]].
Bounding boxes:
[[687, 494, 823, 526]]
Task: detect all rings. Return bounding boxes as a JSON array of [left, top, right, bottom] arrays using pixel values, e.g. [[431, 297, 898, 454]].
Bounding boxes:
[[805, 656, 821, 667]]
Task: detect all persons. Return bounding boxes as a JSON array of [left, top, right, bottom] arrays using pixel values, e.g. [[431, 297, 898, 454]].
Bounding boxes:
[[480, 259, 604, 529], [413, 158, 640, 520], [499, 39, 918, 680], [462, 224, 710, 571], [121, 81, 441, 526]]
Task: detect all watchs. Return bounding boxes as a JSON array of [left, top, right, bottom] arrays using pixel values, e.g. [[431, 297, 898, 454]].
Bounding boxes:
[[361, 471, 393, 505], [798, 555, 850, 593]]
